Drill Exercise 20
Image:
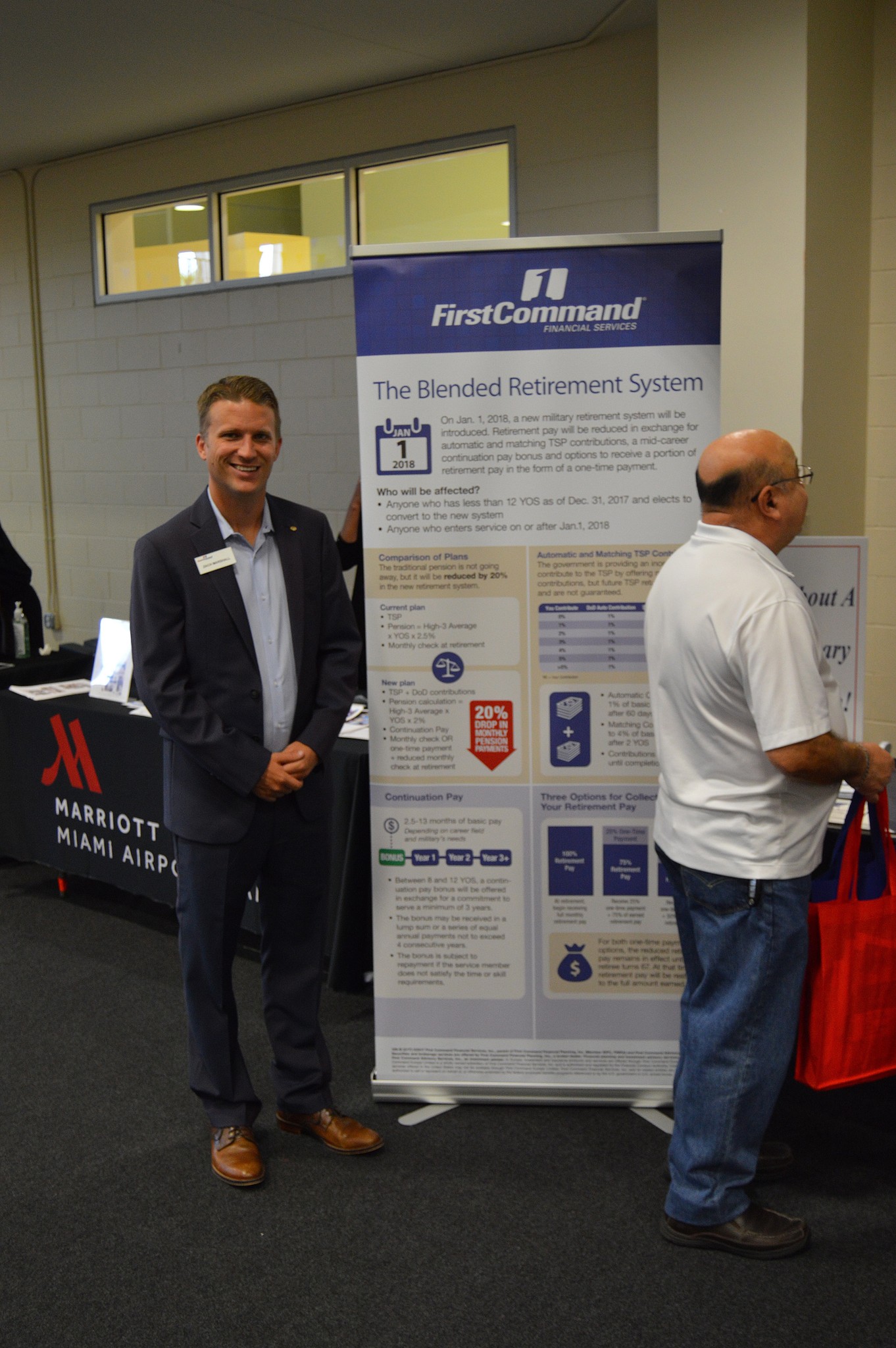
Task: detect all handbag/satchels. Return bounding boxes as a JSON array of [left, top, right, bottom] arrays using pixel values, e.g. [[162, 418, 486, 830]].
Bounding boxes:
[[810, 790, 887, 903], [794, 785, 896, 1092]]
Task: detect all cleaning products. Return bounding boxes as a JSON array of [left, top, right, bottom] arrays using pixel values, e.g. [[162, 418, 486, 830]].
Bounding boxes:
[[12, 601, 30, 659]]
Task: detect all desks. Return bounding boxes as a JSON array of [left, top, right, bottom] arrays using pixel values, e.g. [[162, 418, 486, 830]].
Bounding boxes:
[[1, 670, 896, 1129]]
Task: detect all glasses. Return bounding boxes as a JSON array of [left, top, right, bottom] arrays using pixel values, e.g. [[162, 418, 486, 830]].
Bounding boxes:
[[751, 464, 814, 504]]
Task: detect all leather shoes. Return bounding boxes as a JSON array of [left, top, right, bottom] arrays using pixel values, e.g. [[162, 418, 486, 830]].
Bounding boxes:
[[276, 1102, 384, 1155], [755, 1141, 797, 1181], [208, 1126, 266, 1186], [661, 1203, 811, 1260]]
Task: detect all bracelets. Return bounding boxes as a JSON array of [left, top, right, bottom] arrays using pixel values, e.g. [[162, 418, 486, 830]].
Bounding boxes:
[[848, 742, 871, 787]]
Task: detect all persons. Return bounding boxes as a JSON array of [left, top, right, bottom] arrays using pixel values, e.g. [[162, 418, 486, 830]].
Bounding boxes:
[[129, 377, 386, 1187], [0, 526, 46, 670], [645, 432, 892, 1260]]
